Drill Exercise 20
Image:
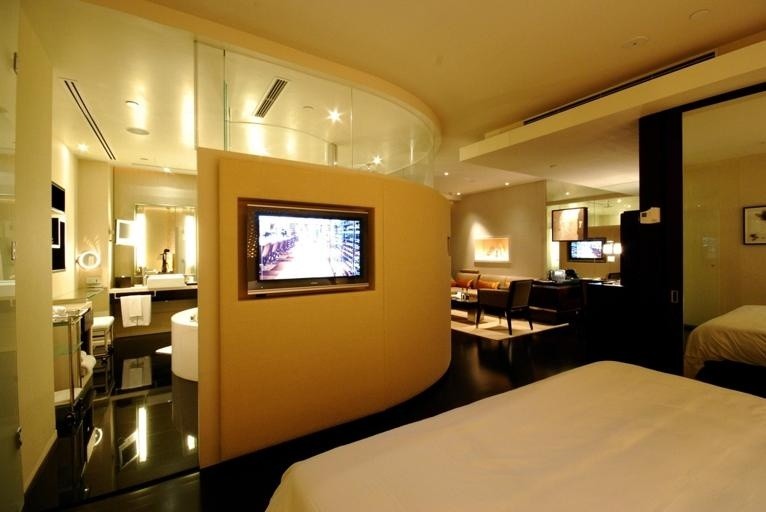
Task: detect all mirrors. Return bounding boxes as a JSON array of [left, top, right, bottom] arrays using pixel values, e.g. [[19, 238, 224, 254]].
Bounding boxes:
[[133, 202, 197, 284]]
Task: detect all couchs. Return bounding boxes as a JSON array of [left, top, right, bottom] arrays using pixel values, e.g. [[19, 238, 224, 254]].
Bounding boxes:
[[450, 268, 538, 295]]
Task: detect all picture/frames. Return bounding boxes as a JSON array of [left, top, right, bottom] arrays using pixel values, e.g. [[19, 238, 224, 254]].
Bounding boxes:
[[742, 204, 766, 245]]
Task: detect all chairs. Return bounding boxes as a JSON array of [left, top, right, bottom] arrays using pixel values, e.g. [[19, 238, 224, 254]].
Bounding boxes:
[[475, 279, 534, 336]]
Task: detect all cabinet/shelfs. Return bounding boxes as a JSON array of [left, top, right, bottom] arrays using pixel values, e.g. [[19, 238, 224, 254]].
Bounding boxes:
[[52, 301, 97, 439]]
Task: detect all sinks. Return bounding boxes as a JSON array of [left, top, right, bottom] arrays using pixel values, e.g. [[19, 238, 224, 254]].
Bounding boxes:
[[147, 274, 185, 285]]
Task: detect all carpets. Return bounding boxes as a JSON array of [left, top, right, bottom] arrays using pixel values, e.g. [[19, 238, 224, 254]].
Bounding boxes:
[[450, 306, 569, 341]]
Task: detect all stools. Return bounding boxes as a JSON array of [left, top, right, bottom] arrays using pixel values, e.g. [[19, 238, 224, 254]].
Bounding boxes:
[[93, 356, 115, 404], [91, 316, 115, 356]]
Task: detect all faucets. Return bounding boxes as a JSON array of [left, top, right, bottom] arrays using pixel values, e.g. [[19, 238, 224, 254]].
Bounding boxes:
[[161, 248, 170, 273]]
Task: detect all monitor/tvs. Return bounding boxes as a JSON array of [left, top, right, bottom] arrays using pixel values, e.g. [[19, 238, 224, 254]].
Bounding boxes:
[[246, 203, 370, 295], [552, 207, 587, 242], [567, 237, 607, 263]]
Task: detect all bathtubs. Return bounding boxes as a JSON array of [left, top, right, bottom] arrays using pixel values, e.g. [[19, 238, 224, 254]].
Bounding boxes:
[[170, 308, 199, 383]]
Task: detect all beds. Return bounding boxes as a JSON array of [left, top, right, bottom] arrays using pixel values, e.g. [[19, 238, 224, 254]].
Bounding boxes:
[[260, 360, 766, 512], [681, 304, 766, 380]]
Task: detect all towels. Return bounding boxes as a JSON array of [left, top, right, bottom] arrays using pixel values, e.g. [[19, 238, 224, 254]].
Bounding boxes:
[[81, 350, 96, 385], [120, 294, 152, 328], [121, 354, 152, 391]]
